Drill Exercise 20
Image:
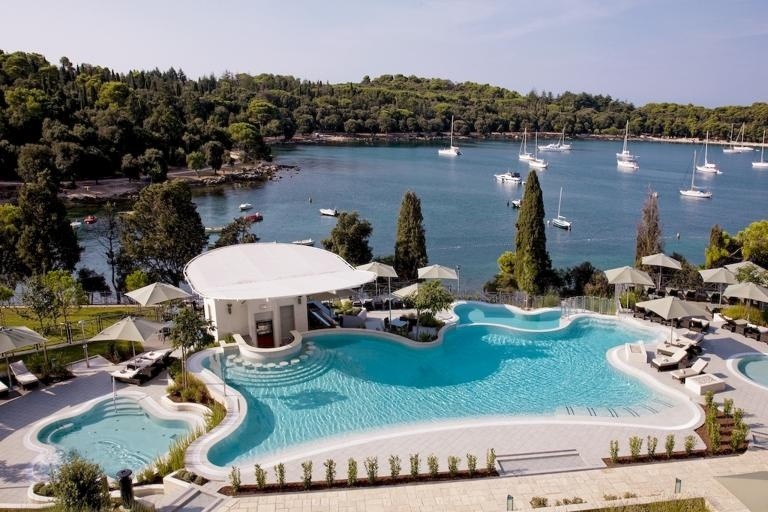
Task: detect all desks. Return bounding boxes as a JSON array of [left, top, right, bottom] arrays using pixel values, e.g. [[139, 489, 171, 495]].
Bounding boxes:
[[389, 319, 407, 336]]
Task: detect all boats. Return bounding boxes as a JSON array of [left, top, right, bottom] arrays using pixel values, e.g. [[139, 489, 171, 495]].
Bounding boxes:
[[494, 171, 522, 185], [291, 237, 314, 245], [319, 208, 339, 217], [83, 215, 97, 224], [510, 199, 521, 208], [69, 221, 81, 229], [239, 203, 251, 209]]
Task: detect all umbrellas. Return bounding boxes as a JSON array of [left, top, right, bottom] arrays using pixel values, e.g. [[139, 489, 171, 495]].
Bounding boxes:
[[417, 263, 458, 281], [634, 294, 713, 346], [0, 324, 48, 391], [697, 260, 768, 321], [87, 314, 164, 369], [642, 250, 684, 291], [354, 261, 399, 297], [604, 264, 656, 312], [124, 281, 193, 323]]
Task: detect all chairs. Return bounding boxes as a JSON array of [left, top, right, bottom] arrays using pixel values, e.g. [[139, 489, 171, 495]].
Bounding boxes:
[[0, 381, 8, 395], [354, 298, 418, 328], [108, 348, 179, 386], [644, 286, 768, 385], [9, 360, 40, 390]]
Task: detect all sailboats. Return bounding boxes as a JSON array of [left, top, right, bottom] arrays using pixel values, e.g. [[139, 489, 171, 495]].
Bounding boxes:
[[722, 122, 767, 167], [438, 115, 461, 156], [615, 119, 639, 161], [551, 186, 570, 231], [678, 130, 717, 199], [518, 127, 571, 168]]
